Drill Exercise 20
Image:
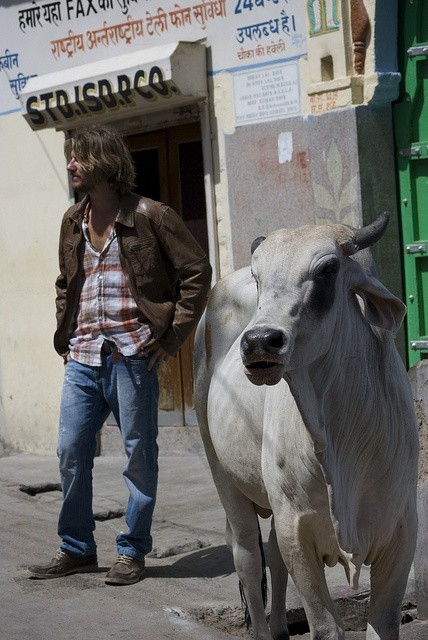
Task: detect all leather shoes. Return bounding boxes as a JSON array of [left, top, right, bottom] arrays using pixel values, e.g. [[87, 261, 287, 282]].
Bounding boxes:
[[104, 554, 144, 584], [27, 549, 98, 579]]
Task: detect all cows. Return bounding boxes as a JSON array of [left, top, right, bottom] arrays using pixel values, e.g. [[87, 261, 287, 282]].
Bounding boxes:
[[191, 211, 418, 640]]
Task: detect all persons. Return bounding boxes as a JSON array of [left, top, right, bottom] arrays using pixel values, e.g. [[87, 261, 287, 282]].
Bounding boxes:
[[22, 122, 212, 585]]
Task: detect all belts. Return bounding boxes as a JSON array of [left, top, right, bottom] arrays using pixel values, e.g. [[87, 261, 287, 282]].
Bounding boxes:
[[101, 341, 117, 354]]
